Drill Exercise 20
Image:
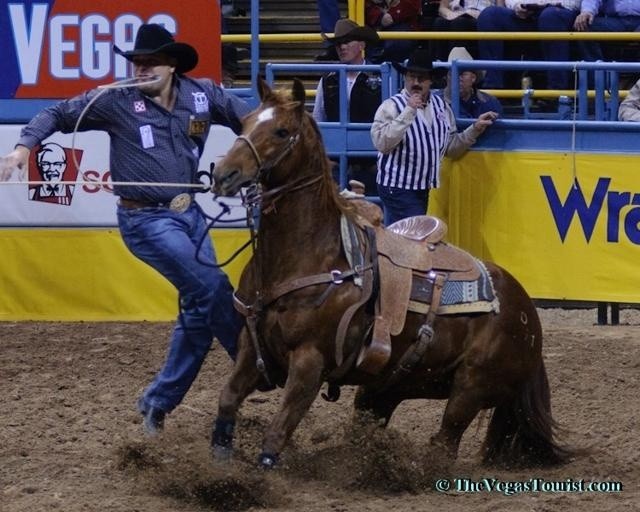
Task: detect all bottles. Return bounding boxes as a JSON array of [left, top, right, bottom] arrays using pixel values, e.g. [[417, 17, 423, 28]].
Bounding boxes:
[[558, 95, 572, 120]]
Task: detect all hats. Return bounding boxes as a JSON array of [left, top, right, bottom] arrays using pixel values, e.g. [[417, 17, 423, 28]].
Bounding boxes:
[[112, 24, 199, 73], [391, 50, 447, 89], [323, 19, 382, 54], [448, 46, 483, 85]]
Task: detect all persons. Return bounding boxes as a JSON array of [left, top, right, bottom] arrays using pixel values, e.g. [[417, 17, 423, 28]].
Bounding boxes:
[[367, 45, 499, 228], [305, 15, 385, 209], [2, 21, 289, 437], [27, 140, 78, 207], [221, 0, 639, 124]]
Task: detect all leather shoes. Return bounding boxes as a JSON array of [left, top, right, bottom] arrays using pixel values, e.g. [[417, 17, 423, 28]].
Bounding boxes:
[[313, 51, 339, 61], [137, 396, 167, 434]]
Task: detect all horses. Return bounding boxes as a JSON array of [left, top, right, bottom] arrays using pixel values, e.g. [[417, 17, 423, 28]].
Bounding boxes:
[[209, 72, 599, 476]]
